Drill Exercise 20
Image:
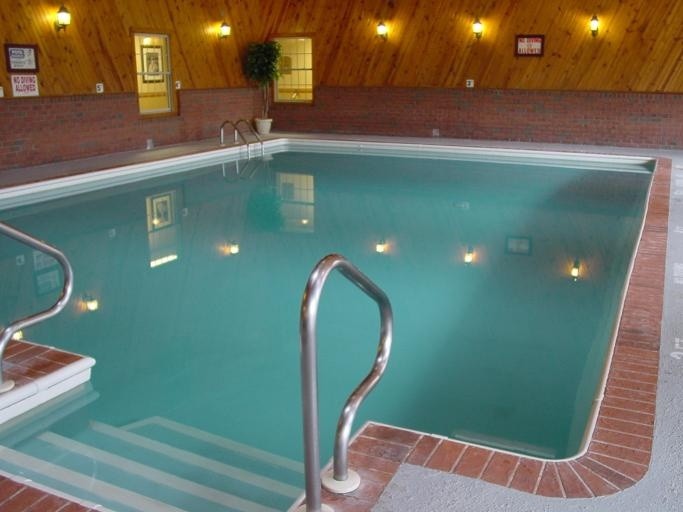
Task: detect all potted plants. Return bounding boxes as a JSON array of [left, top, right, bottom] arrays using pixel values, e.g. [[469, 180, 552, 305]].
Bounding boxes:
[[249, 154, 287, 234], [240, 41, 285, 135]]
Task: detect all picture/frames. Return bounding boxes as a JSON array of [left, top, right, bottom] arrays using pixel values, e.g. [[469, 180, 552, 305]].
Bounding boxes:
[[142, 46, 165, 83], [32, 249, 62, 298], [5, 44, 40, 74], [151, 195, 174, 231], [505, 237, 533, 255], [514, 35, 546, 58]]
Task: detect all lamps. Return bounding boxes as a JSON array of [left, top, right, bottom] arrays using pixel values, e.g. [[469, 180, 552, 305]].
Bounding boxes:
[[227, 240, 240, 255], [219, 21, 232, 42], [377, 15, 599, 42], [375, 237, 583, 279], [56, 4, 72, 33], [83, 293, 100, 313]]
